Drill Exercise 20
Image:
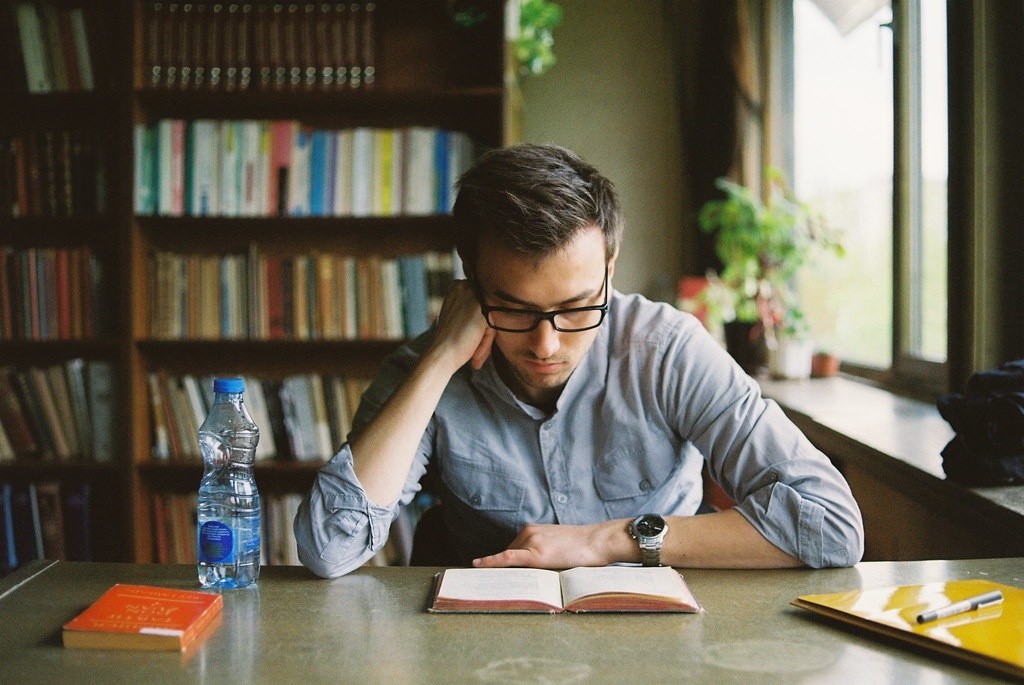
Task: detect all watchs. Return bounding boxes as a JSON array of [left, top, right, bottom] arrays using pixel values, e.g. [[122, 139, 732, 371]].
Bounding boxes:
[[629, 513, 669, 567]]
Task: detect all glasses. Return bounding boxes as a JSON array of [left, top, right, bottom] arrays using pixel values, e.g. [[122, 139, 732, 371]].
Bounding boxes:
[[477, 265, 609, 332]]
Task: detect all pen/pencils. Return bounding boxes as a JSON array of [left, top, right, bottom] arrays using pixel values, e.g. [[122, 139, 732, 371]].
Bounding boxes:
[[915, 590, 1005, 624]]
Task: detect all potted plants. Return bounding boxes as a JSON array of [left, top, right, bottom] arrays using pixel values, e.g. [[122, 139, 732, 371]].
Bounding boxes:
[[697, 163, 857, 380]]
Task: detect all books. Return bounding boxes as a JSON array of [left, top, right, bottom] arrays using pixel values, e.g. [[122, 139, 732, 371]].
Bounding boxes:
[[1, 244, 122, 342], [143, 362, 383, 466], [141, 239, 468, 344], [427, 566, 702, 613], [1, 356, 128, 467], [1, 477, 108, 576], [1, 123, 117, 222], [141, 1, 384, 90], [62, 584, 222, 652], [147, 489, 444, 572], [129, 114, 479, 219], [1, 0, 108, 97]]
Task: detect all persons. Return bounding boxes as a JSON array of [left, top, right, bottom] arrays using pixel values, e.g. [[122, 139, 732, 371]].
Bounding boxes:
[[292, 142, 867, 582]]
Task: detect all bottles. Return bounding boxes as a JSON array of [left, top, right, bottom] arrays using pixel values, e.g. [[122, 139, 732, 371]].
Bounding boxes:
[[197, 377, 262, 589]]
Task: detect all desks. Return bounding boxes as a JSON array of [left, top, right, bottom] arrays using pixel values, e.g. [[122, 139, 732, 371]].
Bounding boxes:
[[0, 557, 1024, 685]]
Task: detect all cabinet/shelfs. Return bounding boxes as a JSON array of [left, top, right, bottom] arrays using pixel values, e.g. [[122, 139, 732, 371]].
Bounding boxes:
[[0, 0, 132, 562], [132, 0, 517, 564]]
